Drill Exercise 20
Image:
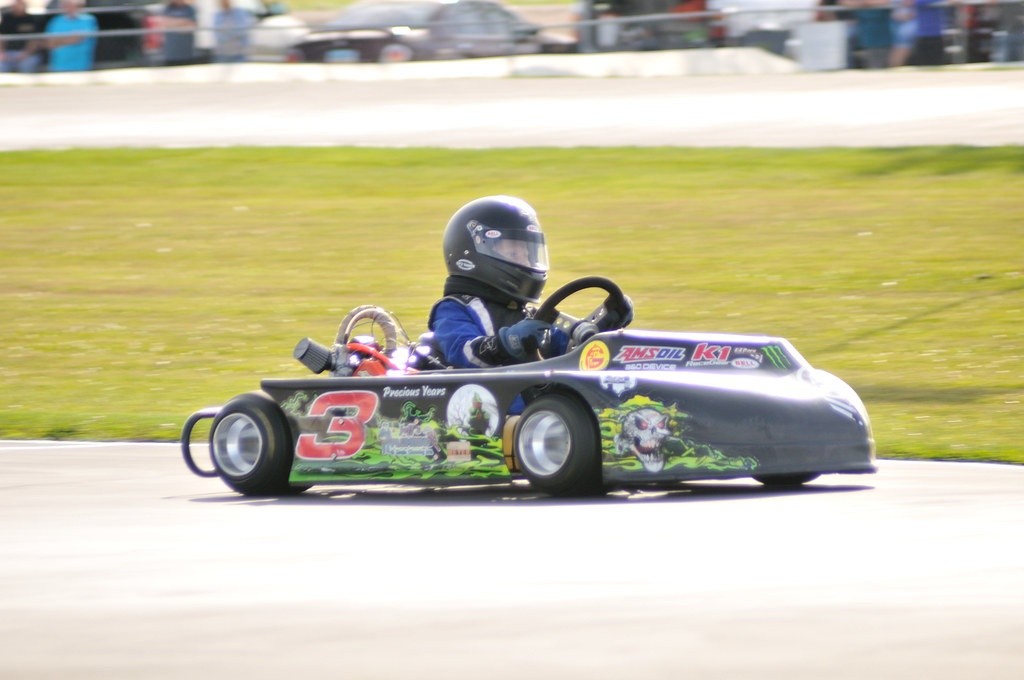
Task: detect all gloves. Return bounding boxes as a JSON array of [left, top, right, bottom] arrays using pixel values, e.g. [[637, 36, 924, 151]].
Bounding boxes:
[[499, 318, 553, 360], [605, 294, 634, 332]]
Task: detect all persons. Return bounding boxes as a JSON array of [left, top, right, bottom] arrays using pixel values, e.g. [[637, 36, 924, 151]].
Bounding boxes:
[[427, 195, 635, 369], [807, 0, 1024, 78], [0, 0, 286, 74]]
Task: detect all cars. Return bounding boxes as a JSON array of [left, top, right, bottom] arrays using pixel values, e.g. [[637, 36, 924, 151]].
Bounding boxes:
[[287, 1, 579, 65], [572, 1, 819, 57]]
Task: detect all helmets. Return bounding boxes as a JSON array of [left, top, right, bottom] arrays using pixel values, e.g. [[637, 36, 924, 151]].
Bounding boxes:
[[444, 195, 549, 305]]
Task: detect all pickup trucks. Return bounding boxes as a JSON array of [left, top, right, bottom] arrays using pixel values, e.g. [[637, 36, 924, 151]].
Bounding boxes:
[[26, 0, 267, 69]]
